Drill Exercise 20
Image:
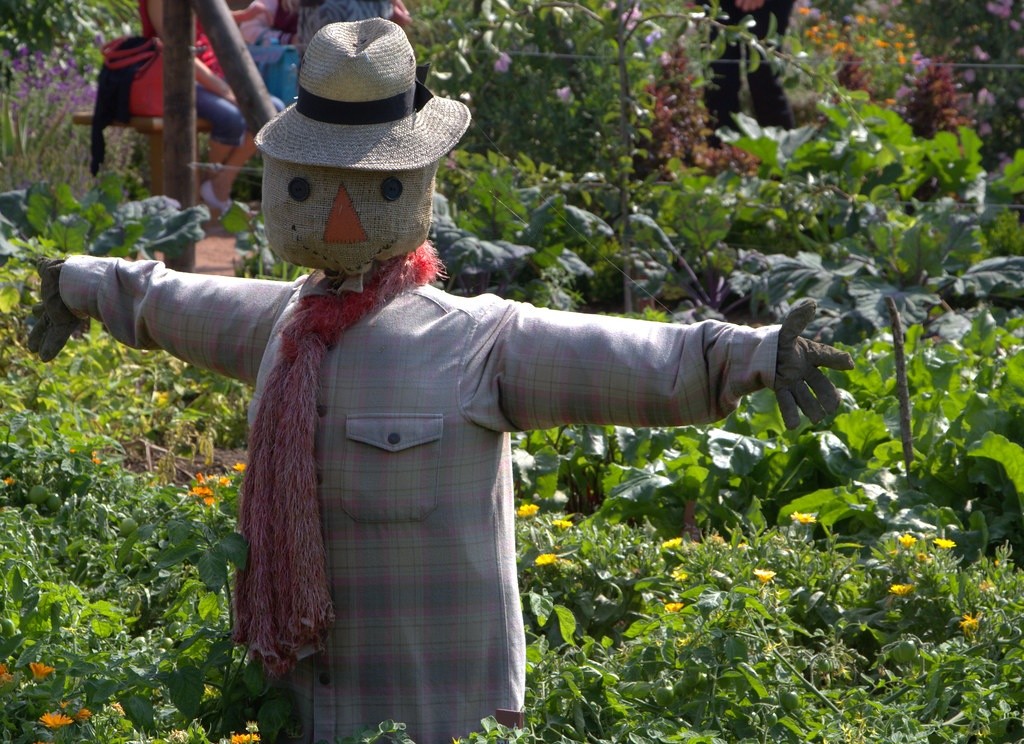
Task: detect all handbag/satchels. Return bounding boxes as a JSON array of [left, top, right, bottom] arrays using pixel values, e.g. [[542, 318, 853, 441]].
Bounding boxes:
[[90, 32, 164, 174]]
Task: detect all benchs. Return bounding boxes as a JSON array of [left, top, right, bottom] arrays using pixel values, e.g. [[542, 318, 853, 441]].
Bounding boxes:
[[73, 110, 214, 198]]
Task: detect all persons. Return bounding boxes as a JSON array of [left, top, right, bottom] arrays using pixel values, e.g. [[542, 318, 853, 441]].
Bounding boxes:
[[132, 1, 413, 218], [693, 1, 793, 150]]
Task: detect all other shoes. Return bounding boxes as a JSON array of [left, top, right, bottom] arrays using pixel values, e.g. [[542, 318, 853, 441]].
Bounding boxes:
[[202, 182, 232, 214]]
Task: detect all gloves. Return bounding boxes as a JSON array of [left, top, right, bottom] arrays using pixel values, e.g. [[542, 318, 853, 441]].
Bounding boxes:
[[769, 294, 857, 431], [24, 256, 83, 364]]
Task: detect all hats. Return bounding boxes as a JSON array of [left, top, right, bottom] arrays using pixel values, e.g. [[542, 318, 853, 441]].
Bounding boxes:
[[252, 17, 474, 172]]
